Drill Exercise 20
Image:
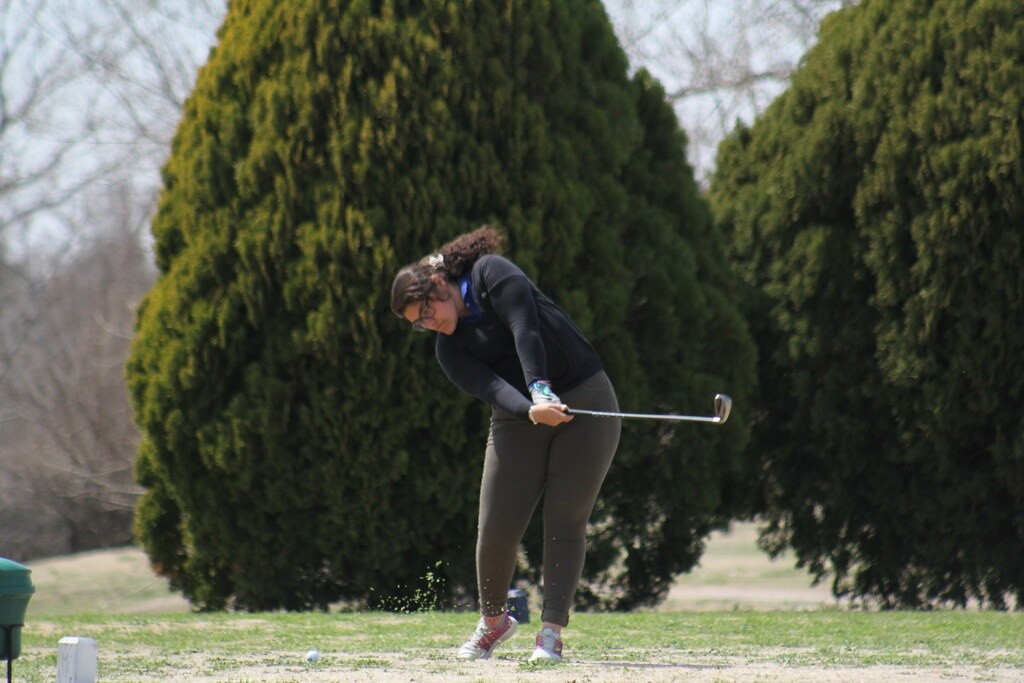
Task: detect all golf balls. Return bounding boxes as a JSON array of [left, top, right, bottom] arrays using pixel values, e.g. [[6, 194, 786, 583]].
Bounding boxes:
[[306, 649, 320, 664]]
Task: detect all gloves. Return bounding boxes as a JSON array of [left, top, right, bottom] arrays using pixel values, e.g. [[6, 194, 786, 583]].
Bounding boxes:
[[529, 382, 560, 407]]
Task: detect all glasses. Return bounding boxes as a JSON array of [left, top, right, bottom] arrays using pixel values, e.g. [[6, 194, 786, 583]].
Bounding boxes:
[[410, 297, 436, 332]]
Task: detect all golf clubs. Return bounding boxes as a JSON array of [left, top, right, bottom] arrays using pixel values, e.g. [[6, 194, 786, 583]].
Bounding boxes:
[[563, 394, 733, 425]]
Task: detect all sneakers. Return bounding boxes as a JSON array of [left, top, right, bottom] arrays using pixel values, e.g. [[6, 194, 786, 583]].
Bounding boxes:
[[529, 629, 564, 663], [455, 615, 519, 659]]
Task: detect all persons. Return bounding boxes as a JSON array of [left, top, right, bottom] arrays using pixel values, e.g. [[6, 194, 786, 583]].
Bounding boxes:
[[389, 223, 621, 666]]
